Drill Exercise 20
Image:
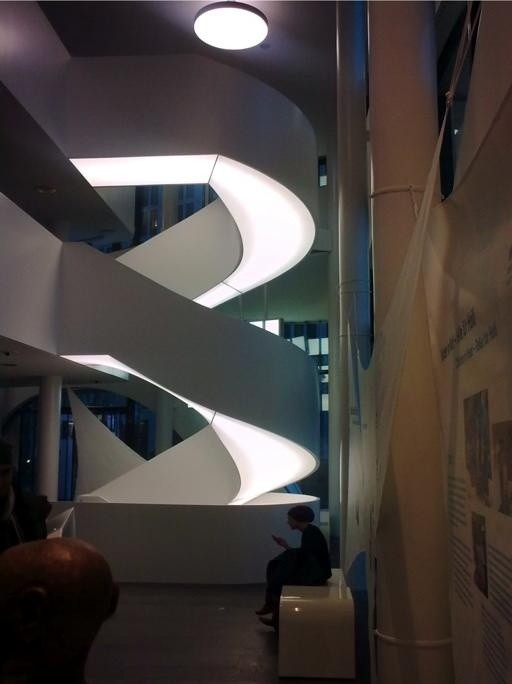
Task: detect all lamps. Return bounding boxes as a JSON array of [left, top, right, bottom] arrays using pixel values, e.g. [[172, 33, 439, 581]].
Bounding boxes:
[[193, 2, 268, 51]]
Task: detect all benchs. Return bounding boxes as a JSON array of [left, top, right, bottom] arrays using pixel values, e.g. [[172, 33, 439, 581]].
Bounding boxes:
[[278, 568, 356, 678]]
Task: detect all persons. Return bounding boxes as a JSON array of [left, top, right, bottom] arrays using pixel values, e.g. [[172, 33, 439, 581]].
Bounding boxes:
[[256, 505, 333, 631]]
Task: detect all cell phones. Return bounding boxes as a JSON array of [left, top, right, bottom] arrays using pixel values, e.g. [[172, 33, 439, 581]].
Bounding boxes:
[[271, 534, 280, 544]]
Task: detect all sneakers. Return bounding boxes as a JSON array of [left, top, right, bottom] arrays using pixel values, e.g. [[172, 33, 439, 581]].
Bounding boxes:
[[254, 603, 273, 615], [258, 616, 274, 626]]
